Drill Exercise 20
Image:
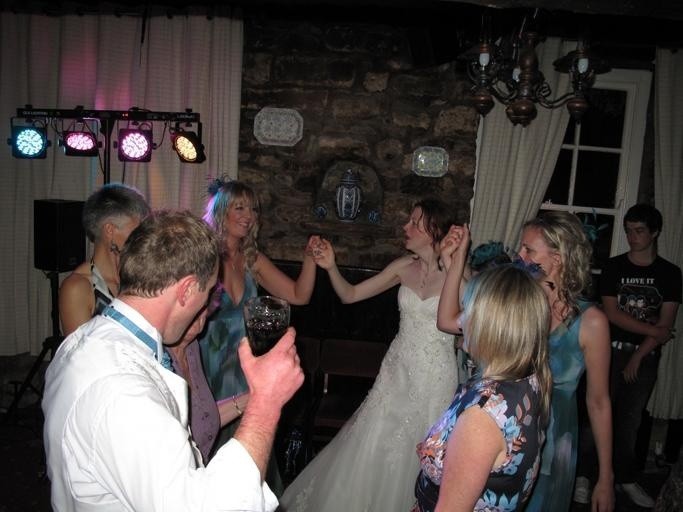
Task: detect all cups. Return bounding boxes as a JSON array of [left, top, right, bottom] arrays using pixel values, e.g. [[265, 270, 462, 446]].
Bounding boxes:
[[242, 295, 292, 357]]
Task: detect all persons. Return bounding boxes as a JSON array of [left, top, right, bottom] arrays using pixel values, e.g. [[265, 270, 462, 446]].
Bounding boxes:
[[60, 185, 152, 337], [163, 285, 251, 468], [415, 265, 550, 512], [573, 205, 681, 511], [202, 181, 321, 495], [41, 210, 305, 510], [280, 196, 461, 512], [436, 208, 614, 512]]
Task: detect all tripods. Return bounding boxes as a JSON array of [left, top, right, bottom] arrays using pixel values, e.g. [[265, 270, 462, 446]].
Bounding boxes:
[[2, 273, 69, 421]]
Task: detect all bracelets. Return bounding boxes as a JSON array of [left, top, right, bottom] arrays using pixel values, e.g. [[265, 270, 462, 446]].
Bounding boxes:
[[231, 397, 244, 414]]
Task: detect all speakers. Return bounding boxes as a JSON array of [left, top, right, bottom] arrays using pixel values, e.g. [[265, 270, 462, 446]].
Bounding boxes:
[[35, 199, 87, 272]]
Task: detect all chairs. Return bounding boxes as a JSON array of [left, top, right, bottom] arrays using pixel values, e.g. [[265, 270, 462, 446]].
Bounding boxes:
[[281, 332, 324, 450], [312, 337, 386, 449]]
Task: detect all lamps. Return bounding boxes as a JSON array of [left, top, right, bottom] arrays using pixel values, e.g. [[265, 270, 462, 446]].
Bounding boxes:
[[56, 119, 104, 157], [170, 119, 207, 164], [112, 119, 159, 163], [6, 114, 53, 159], [465, 8, 606, 129]]
